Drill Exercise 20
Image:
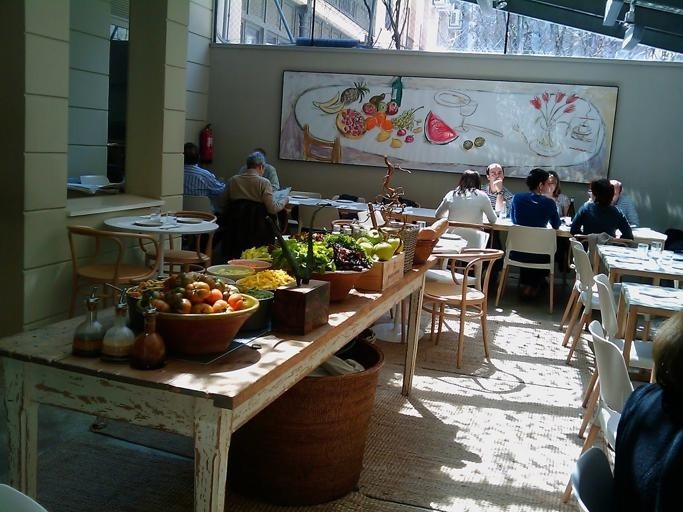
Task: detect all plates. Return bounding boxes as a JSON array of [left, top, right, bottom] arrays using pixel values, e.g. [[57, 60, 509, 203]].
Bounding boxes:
[[337, 200, 353, 203], [177, 217, 202, 224], [294, 195, 308, 199], [135, 220, 163, 226]]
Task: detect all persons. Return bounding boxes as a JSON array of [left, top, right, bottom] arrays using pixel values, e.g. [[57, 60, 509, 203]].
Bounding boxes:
[[509, 168, 562, 304], [238, 147, 280, 192], [612, 311, 683, 512], [569, 177, 634, 241], [547, 170, 573, 289], [481, 163, 514, 281], [184, 143, 225, 213], [610, 180, 639, 228], [434, 168, 498, 231], [217, 151, 289, 214]]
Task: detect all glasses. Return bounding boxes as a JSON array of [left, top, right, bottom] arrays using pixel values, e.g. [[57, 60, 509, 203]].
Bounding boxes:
[[588, 190, 592, 194]]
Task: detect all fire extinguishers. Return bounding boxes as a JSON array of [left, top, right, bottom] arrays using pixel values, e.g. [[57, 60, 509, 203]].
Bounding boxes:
[[199, 123, 214, 164]]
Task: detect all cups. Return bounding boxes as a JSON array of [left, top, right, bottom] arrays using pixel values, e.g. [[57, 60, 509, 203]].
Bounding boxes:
[[166, 208, 176, 225], [417, 220, 426, 229], [151, 207, 161, 222], [637, 243, 648, 259], [651, 242, 662, 257]]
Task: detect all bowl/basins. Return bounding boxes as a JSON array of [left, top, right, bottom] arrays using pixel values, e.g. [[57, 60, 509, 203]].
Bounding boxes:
[[228, 258, 271, 271], [135, 291, 259, 356], [313, 265, 368, 306], [206, 265, 254, 279]]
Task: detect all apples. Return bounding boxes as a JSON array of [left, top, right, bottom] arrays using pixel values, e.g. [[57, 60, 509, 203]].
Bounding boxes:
[[356, 236, 368, 244], [390, 237, 404, 252], [376, 241, 393, 261], [359, 242, 373, 256], [369, 228, 379, 239]]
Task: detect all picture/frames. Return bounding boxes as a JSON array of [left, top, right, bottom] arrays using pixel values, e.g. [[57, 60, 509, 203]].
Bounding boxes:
[[277, 69, 620, 185]]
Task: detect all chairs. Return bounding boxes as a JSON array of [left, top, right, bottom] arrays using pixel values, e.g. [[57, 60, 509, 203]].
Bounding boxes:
[[382, 197, 421, 208], [568, 233, 634, 270], [562, 243, 650, 365], [330, 219, 354, 230], [562, 320, 635, 505], [448, 220, 493, 248], [444, 226, 490, 293], [183, 195, 212, 214], [290, 190, 321, 199], [558, 237, 602, 330], [576, 273, 656, 438], [421, 247, 504, 370], [331, 194, 366, 203], [138, 210, 217, 275], [65, 224, 161, 320], [494, 224, 558, 315]]
